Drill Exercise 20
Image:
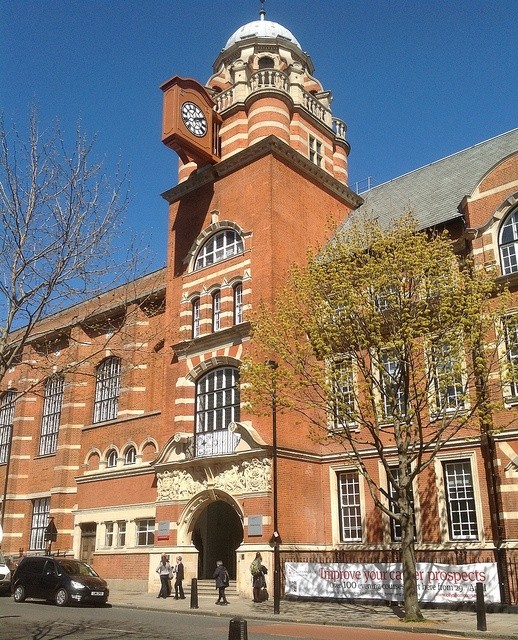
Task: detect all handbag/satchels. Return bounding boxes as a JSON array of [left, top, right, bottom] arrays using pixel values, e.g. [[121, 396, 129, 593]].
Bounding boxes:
[[169, 570, 173, 580]]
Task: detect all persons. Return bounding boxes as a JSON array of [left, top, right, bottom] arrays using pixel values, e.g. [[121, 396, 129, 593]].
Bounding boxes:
[[250, 552, 268, 603], [211, 560, 230, 606], [156, 552, 174, 599], [173, 555, 186, 600]]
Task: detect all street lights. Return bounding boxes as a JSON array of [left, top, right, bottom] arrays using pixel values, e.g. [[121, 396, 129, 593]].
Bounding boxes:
[[265, 360, 281, 614]]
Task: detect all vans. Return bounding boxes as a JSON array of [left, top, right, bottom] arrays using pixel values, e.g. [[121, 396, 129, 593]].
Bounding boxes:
[[11, 556, 109, 607]]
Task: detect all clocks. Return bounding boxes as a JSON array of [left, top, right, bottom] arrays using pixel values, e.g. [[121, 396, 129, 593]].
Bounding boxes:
[[181, 101, 208, 137]]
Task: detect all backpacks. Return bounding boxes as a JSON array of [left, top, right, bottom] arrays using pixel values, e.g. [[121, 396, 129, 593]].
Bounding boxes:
[[222, 569, 230, 583], [250, 560, 260, 576]]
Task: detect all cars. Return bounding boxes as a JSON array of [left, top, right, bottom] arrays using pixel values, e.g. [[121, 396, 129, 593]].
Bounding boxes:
[[0, 551, 12, 596]]
